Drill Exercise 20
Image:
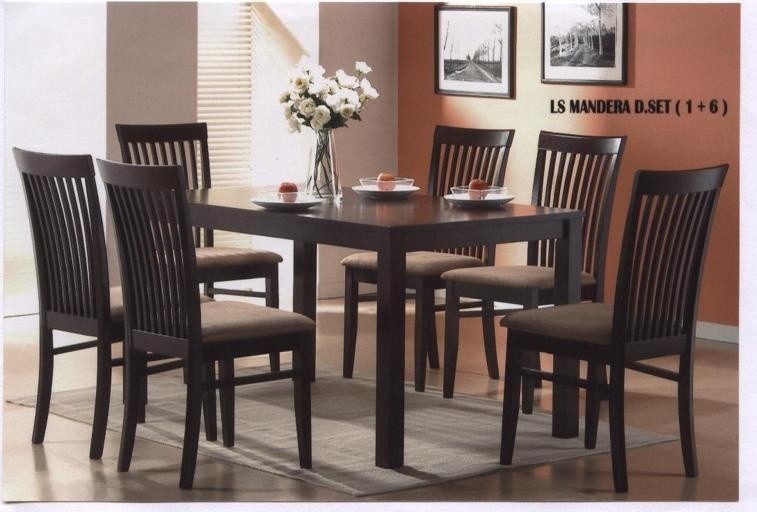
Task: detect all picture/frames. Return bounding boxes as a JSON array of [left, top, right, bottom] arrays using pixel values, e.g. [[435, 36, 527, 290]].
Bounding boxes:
[[431, 4, 513, 99], [540, 3, 626, 85]]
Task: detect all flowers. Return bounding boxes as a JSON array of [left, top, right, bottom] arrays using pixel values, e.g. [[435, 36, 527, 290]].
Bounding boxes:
[[278, 54, 380, 199]]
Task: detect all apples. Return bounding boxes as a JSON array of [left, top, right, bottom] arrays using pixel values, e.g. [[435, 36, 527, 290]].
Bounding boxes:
[[278, 180, 296, 201], [468, 180, 489, 199], [378, 173, 396, 189]]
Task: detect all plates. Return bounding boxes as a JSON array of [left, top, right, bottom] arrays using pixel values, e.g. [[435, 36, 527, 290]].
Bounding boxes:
[[441, 187, 518, 210], [248, 191, 330, 211], [353, 178, 422, 197]]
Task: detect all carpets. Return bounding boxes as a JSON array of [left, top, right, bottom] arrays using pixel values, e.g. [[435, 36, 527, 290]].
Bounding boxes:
[[7, 358, 681, 496]]
